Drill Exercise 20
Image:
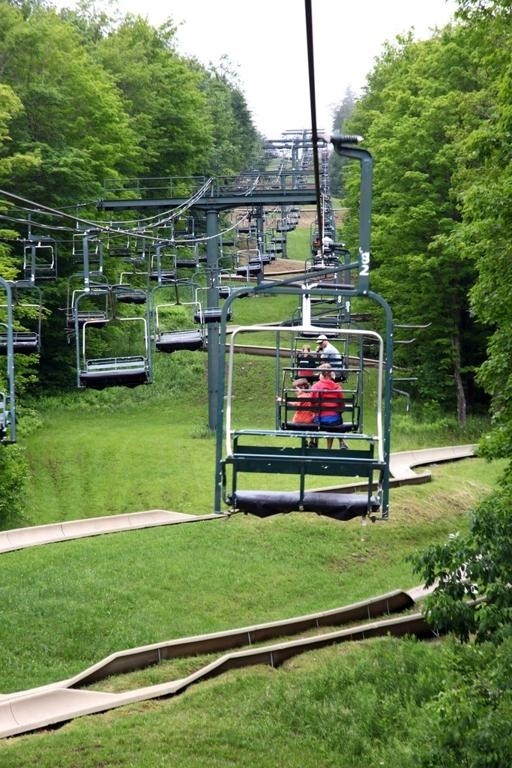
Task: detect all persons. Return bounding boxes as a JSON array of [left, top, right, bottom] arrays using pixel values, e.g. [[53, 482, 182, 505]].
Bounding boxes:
[[313, 236, 333, 267], [316, 335, 346, 383], [296, 345, 316, 387], [310, 363, 348, 449], [276, 378, 316, 448]]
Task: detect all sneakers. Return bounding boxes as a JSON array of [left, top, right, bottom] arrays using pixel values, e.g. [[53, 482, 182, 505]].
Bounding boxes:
[[340, 443, 348, 449], [302, 441, 316, 450]]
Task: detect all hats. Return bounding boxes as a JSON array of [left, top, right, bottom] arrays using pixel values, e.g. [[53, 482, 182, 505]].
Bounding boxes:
[[316, 335, 328, 344], [293, 377, 307, 386]]
